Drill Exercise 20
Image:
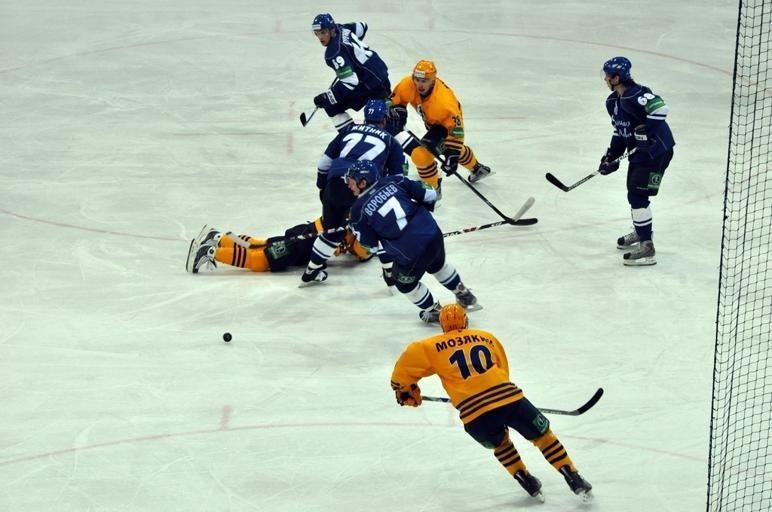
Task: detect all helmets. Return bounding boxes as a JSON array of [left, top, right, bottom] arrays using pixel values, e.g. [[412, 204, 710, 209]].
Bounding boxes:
[[346, 160, 381, 186], [412, 60, 437, 82], [600, 57, 631, 86], [364, 100, 391, 123], [311, 13, 337, 40], [439, 304, 469, 333]]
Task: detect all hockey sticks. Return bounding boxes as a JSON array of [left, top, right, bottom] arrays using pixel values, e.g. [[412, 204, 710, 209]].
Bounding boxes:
[[225, 224, 355, 249], [441, 218, 538, 238], [405, 126, 536, 224], [299, 78, 338, 126], [421, 388, 603, 416], [545, 149, 638, 193]]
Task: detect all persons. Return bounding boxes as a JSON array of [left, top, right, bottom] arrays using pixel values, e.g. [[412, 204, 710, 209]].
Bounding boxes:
[[311, 13, 421, 156], [302, 99, 403, 287], [391, 304, 592, 498], [388, 60, 490, 201], [193, 215, 375, 273], [599, 56, 676, 260], [346, 159, 477, 323]]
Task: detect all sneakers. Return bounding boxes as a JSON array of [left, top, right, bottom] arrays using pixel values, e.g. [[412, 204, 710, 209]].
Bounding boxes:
[[382, 268, 395, 287], [624, 241, 655, 260], [514, 468, 542, 497], [191, 224, 224, 273], [457, 283, 477, 308], [617, 227, 653, 247], [559, 464, 592, 495], [301, 270, 327, 283], [468, 164, 491, 182], [418, 301, 441, 322]]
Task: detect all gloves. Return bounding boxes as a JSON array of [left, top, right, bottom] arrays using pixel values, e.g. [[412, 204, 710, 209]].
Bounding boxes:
[[441, 149, 460, 177], [634, 124, 656, 149], [599, 148, 623, 175], [391, 383, 422, 407], [392, 104, 407, 132], [314, 87, 343, 107]]
